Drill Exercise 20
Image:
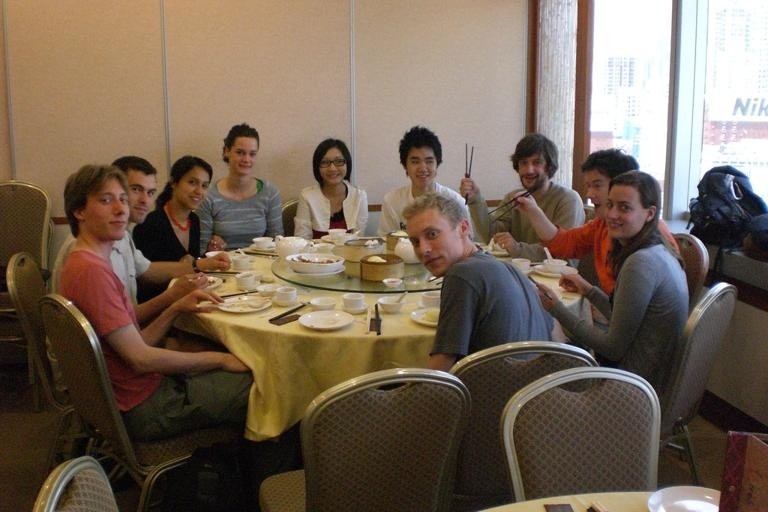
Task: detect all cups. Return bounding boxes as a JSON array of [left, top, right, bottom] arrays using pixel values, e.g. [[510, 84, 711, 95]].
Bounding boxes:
[[513, 258, 531, 271]]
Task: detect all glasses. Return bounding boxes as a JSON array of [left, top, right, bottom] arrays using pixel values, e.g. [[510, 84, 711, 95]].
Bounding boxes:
[[320, 158, 346, 168]]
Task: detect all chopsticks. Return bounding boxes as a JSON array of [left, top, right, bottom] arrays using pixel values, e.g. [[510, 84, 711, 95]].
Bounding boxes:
[[527, 275, 554, 301], [488, 183, 541, 226], [487, 251, 510, 258], [464, 144, 474, 205]]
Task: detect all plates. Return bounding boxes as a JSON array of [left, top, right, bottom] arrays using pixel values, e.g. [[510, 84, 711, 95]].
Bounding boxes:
[[194, 232, 444, 331], [535, 264, 579, 277], [647, 483, 722, 510]]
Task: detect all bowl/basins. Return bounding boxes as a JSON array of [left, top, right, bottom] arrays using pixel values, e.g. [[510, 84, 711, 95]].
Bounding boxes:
[[543, 259, 568, 273], [329, 229, 346, 240]]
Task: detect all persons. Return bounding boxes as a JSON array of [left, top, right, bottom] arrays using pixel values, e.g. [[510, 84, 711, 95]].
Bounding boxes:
[[293, 139, 369, 240]]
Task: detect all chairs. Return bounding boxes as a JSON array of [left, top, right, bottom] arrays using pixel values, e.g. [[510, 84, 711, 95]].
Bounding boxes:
[[6, 251, 76, 480], [448, 342, 598, 512], [583, 205, 595, 215], [0, 180, 54, 412], [661, 282, 738, 485], [259, 367, 472, 512], [499, 365, 662, 502], [32, 457, 119, 512], [281, 198, 297, 233], [34, 294, 224, 512], [673, 233, 710, 311]]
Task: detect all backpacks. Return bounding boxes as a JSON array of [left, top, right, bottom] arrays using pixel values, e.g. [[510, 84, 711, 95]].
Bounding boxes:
[[686, 165, 768, 264]]
